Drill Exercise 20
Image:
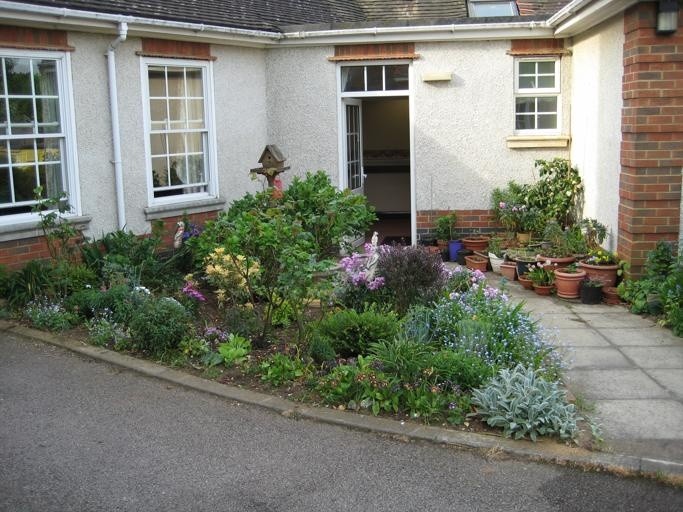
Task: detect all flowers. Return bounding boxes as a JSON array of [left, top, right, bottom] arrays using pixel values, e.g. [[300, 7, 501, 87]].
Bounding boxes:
[[431, 157, 624, 306]]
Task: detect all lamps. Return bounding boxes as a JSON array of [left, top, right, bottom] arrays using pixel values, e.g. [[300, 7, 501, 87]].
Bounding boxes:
[[655, 0, 678, 36]]
